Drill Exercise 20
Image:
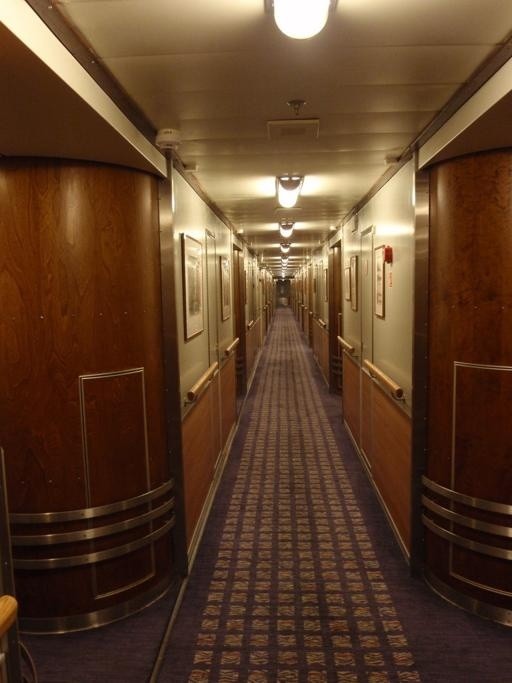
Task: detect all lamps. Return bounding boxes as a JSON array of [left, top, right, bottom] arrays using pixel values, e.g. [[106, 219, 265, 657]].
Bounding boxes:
[[276, 173, 305, 268], [264, 0, 338, 42]]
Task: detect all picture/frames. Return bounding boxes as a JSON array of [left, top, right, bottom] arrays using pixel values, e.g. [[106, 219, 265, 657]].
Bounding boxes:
[[344, 245, 385, 319], [181, 232, 231, 343]]
